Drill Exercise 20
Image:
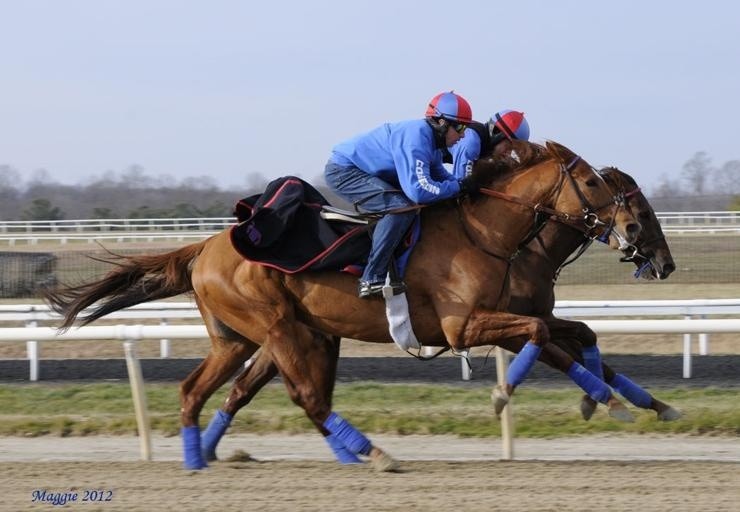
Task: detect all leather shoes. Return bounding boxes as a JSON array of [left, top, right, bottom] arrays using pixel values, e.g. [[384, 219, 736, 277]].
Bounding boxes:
[[358, 280, 408, 299]]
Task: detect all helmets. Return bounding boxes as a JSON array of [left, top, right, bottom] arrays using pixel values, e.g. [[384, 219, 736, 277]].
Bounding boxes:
[[492, 110, 530, 144], [425, 91, 471, 124]]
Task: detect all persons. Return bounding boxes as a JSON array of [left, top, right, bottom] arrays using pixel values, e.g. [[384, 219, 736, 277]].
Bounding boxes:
[[324, 88, 490, 301], [441, 110, 532, 205]]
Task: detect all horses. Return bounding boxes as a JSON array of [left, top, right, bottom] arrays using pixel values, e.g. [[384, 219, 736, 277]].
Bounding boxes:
[[75, 166, 684, 465], [39, 139, 643, 479]]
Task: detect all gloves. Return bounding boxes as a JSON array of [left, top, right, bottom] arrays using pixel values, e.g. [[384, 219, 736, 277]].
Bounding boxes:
[[458, 175, 480, 192]]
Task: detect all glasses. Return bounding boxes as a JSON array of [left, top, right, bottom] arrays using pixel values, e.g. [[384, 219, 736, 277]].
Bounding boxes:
[[453, 123, 467, 132]]
